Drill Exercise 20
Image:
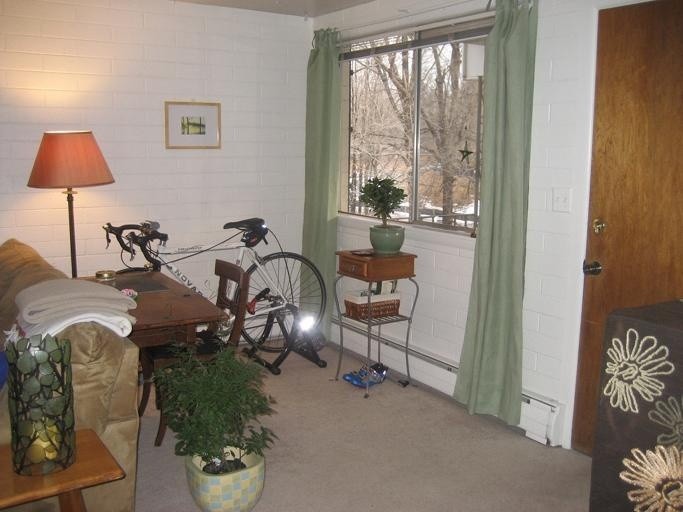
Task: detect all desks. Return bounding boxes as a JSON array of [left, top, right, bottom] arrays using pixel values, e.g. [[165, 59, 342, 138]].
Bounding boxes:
[[78, 272, 229, 446]]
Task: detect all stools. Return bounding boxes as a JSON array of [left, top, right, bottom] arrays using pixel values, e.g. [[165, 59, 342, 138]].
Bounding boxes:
[[0, 427, 124, 511], [137, 259, 250, 447]]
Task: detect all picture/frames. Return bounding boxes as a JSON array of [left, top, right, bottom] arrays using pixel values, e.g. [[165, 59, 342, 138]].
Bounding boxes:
[[162, 102, 221, 150]]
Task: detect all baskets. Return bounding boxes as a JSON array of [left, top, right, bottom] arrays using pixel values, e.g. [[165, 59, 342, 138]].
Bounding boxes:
[[343, 298, 400, 319]]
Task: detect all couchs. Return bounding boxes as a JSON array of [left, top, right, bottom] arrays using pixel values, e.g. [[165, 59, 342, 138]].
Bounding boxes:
[[0, 239, 137, 512]]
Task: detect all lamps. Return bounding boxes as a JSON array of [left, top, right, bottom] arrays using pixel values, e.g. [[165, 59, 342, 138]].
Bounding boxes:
[[26, 130, 114, 275]]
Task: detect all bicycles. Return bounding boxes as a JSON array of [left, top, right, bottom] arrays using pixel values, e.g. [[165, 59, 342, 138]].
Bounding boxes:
[[102, 217, 327, 353]]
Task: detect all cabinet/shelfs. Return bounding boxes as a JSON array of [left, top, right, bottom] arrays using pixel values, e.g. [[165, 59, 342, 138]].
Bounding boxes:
[[330, 250, 418, 398]]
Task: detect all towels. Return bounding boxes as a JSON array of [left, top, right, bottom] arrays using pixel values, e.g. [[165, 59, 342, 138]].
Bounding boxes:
[[9, 277, 138, 340]]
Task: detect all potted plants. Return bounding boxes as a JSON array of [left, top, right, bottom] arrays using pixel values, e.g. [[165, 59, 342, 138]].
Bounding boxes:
[[147, 326, 280, 511], [360, 179, 405, 254]]
